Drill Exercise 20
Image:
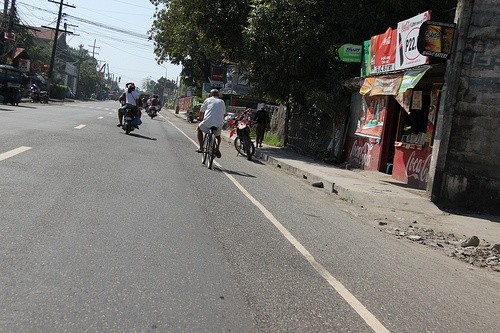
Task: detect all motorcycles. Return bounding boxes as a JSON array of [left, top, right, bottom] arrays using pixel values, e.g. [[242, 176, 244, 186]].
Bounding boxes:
[[229, 108, 256, 161]]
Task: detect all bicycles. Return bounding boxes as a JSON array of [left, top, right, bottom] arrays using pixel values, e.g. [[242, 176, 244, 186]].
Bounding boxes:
[[197, 117, 216, 169]]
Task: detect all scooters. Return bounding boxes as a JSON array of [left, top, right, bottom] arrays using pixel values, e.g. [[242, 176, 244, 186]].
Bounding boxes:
[[143, 101, 157, 119], [30, 83, 49, 104], [119, 99, 138, 134]]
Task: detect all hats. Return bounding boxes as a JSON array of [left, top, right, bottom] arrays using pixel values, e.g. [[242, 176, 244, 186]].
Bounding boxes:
[[260, 103, 265, 108], [128, 84, 134, 88]]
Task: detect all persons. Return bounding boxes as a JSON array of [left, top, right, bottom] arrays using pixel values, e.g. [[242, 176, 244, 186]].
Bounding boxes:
[[195, 89, 227, 158], [250, 102, 271, 148], [117, 83, 162, 127]]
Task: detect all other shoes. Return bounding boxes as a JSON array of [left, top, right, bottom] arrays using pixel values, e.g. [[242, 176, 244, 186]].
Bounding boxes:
[[195, 149, 204, 152], [216, 151, 221, 158], [116, 123, 122, 127]]
